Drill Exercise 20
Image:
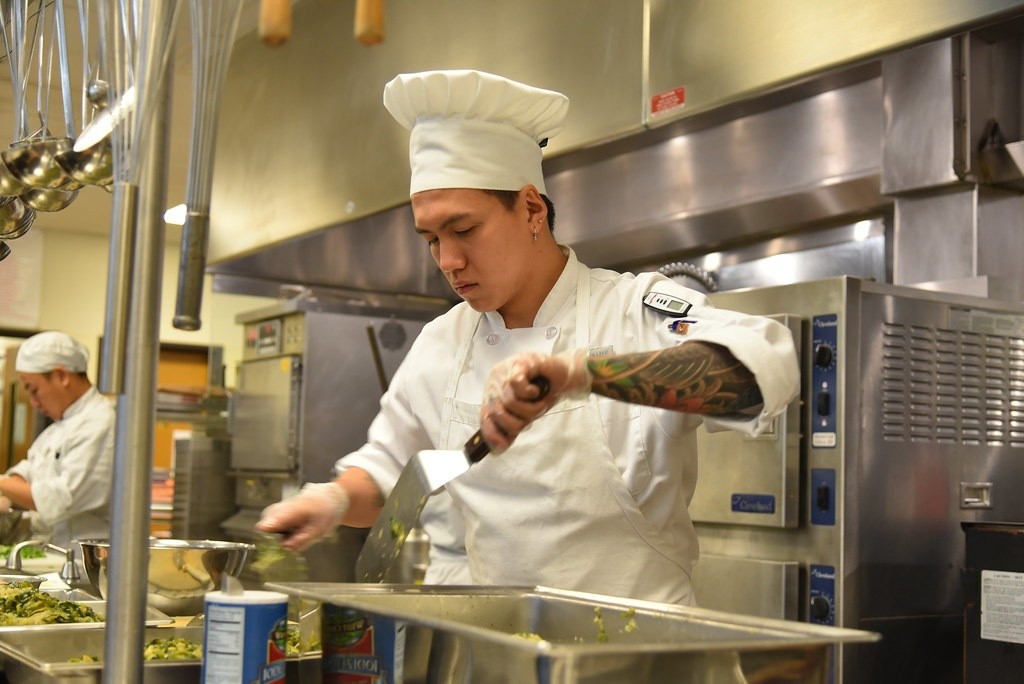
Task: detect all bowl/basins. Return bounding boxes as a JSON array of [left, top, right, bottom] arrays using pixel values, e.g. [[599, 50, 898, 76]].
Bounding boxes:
[[0, 508, 34, 546], [73, 537, 257, 617]]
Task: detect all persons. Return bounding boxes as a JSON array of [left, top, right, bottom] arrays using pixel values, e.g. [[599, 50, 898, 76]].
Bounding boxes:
[[0, 331, 116, 559], [256, 69, 801, 609]]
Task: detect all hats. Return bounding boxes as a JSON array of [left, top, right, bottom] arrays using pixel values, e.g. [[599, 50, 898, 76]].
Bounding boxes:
[[15, 331, 89, 373], [383, 69, 570, 197]]
